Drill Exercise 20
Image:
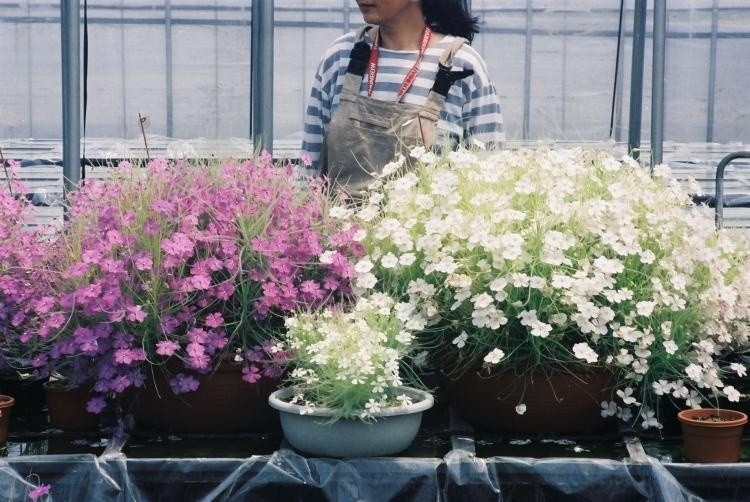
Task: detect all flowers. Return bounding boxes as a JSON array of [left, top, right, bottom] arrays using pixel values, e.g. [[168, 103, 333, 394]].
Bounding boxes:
[[0, 152, 63, 386], [286, 292, 427, 424], [153, 143, 365, 393], [50, 158, 161, 415]]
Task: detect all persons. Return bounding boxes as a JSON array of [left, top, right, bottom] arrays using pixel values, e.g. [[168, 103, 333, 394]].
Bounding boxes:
[[296, 0, 504, 210]]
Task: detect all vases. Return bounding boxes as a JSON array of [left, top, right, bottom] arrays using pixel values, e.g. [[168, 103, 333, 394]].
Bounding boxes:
[[460, 369, 643, 436], [677, 408, 747, 464], [45, 376, 101, 428], [4, 376, 43, 409], [153, 354, 266, 433], [0, 393, 14, 445], [269, 382, 436, 457]]
